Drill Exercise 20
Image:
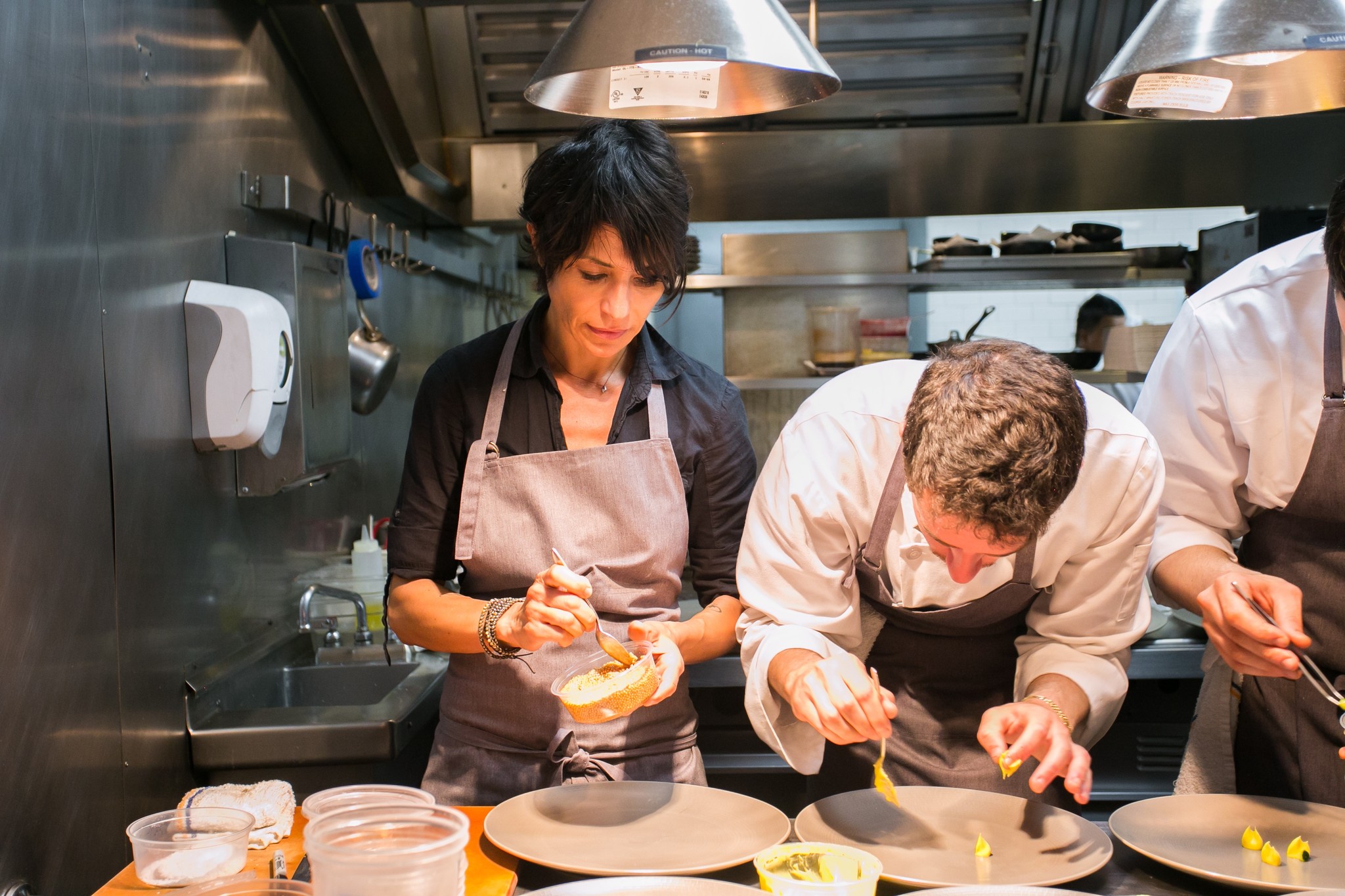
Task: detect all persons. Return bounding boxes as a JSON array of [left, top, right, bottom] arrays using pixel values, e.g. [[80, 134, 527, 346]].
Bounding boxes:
[[735, 339, 1166, 820], [1133, 179, 1345, 809], [1073, 294, 1145, 413], [386, 118, 756, 806]]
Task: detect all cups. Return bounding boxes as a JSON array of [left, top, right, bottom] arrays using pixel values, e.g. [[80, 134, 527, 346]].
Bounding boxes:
[[302, 803, 470, 896], [809, 306, 861, 365], [301, 784, 436, 832]]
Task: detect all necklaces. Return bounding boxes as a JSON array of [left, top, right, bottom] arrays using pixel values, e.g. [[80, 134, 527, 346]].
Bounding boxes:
[[540, 337, 629, 392]]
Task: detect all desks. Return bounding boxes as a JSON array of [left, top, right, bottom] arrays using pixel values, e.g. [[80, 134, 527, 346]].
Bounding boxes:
[[91, 798, 1248, 896]]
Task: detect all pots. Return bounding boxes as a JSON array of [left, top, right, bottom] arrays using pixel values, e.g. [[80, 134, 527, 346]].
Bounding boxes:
[[348, 298, 400, 416]]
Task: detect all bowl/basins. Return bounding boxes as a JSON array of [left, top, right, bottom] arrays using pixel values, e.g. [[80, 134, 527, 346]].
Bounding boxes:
[[933, 222, 1124, 256], [1048, 351, 1102, 370], [1126, 246, 1188, 266], [551, 640, 660, 724], [192, 879, 313, 896], [126, 806, 256, 887], [295, 564, 390, 631], [754, 842, 883, 896]]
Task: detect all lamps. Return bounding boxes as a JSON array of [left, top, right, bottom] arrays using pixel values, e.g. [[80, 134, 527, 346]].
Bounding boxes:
[[1068, 0, 1345, 132], [511, 1, 846, 130]]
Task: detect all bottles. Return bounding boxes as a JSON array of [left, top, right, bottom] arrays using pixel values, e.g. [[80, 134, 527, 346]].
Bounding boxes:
[[351, 525, 383, 576]]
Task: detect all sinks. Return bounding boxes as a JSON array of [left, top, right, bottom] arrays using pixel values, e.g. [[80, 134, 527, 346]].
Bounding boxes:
[[286, 644, 451, 673], [186, 662, 445, 733]]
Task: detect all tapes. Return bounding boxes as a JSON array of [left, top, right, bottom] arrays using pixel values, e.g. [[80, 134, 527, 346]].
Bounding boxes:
[[348, 237, 382, 298]]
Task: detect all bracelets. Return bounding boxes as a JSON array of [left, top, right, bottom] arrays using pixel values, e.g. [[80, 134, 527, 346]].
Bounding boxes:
[[1019, 695, 1071, 735], [478, 596, 536, 676]]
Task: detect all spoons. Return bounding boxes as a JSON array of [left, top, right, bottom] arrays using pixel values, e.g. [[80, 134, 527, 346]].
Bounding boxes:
[[551, 548, 633, 666], [870, 667, 901, 807]]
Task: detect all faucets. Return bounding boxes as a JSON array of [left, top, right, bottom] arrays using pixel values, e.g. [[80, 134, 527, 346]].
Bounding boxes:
[[300, 584, 371, 643]]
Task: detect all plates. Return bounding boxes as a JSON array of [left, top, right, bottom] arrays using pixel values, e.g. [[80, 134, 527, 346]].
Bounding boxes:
[[794, 786, 1114, 887], [519, 876, 776, 896], [897, 887, 1105, 896], [484, 781, 792, 876], [1279, 891, 1345, 896], [1108, 794, 1345, 893]]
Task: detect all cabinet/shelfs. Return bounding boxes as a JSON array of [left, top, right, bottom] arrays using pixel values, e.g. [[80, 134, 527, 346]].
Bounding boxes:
[[644, 247, 1208, 398]]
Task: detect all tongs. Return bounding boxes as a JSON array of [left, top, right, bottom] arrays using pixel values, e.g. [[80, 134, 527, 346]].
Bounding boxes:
[[1231, 582, 1345, 705]]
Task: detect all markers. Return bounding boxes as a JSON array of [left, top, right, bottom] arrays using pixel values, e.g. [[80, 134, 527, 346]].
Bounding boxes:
[[272, 849, 289, 892]]
